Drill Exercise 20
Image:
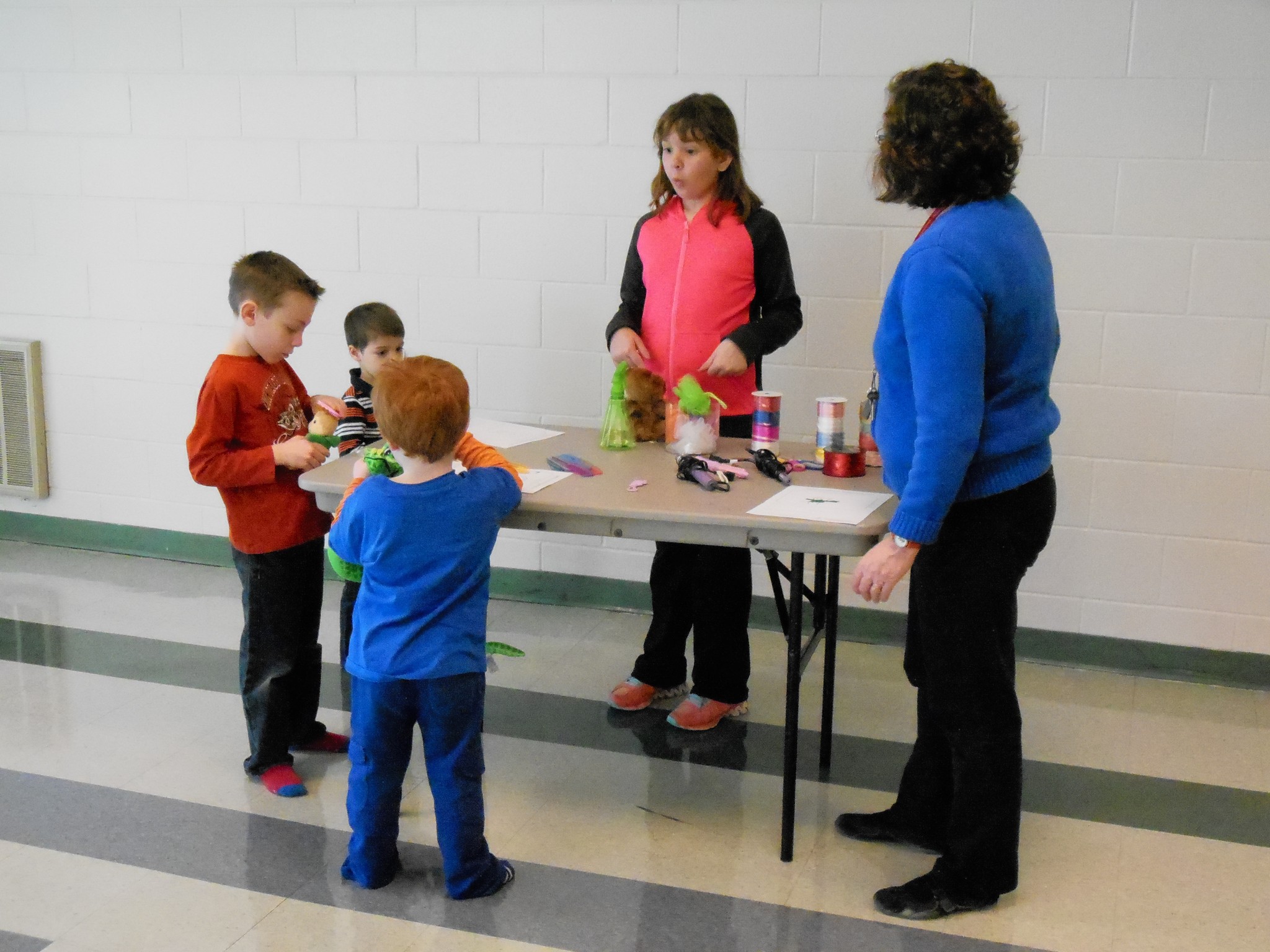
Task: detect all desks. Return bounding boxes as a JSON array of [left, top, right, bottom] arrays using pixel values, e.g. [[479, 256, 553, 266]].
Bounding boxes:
[[303, 419, 902, 861]]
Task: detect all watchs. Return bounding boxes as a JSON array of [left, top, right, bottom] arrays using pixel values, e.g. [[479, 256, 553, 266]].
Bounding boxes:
[[891, 533, 923, 550]]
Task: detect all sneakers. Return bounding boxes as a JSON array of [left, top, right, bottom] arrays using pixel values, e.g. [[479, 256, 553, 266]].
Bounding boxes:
[[873, 871, 999, 920], [835, 808, 952, 854], [667, 695, 750, 730], [608, 677, 689, 710]]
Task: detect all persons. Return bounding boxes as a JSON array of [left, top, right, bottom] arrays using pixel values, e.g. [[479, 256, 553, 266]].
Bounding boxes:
[[324, 352, 524, 901], [603, 92, 805, 732], [185, 249, 353, 801], [325, 301, 407, 665], [828, 58, 1059, 918]]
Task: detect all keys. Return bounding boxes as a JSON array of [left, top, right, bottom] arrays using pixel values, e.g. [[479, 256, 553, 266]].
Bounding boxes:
[[865, 372, 880, 420]]
